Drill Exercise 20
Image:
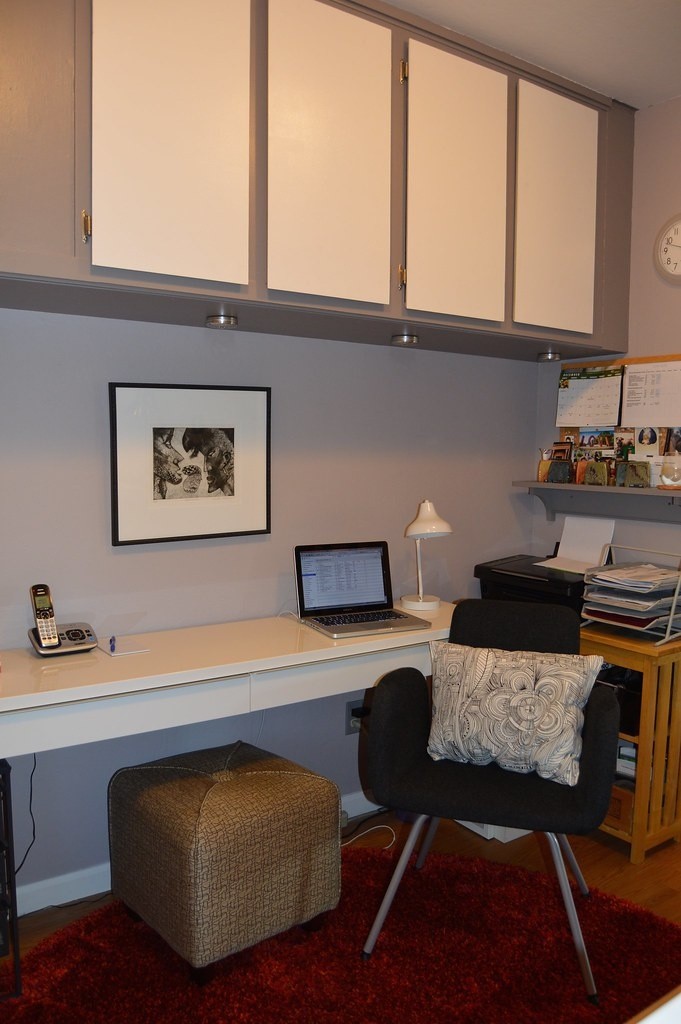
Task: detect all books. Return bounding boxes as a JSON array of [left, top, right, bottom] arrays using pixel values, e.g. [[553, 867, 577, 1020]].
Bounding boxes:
[[585, 564, 681, 628]]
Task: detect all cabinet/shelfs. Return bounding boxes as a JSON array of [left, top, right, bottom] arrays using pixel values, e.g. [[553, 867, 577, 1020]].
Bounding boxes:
[[579, 542, 681, 646], [579, 622, 681, 865], [0, 0, 639, 362]]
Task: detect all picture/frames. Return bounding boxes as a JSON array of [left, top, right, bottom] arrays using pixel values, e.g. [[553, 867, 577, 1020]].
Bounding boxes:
[[108, 381, 273, 546]]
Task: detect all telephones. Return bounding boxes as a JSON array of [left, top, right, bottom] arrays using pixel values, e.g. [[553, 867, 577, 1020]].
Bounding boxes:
[[28, 584, 98, 658]]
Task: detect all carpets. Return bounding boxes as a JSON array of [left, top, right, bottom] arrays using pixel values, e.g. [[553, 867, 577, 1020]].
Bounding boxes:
[[0, 848, 681, 1024]]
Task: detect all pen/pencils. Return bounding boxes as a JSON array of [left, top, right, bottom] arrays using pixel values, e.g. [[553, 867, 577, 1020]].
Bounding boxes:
[[109, 635, 116, 652]]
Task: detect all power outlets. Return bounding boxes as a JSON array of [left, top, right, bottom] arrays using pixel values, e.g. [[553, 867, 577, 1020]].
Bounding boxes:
[[345, 698, 362, 736]]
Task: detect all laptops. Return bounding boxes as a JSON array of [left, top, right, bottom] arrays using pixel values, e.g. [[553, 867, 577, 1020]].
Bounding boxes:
[[294, 541, 432, 639]]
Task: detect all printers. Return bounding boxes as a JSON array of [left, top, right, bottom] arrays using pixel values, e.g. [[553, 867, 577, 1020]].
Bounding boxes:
[[473, 542, 613, 624]]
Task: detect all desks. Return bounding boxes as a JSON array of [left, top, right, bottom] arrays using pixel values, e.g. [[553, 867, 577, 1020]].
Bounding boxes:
[[0, 602, 458, 995]]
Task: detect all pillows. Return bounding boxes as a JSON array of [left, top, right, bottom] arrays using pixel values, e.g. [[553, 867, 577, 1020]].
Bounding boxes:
[[428, 640, 605, 786]]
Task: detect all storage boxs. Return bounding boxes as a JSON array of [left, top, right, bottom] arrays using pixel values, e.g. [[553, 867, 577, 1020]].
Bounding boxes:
[[604, 778, 636, 836]]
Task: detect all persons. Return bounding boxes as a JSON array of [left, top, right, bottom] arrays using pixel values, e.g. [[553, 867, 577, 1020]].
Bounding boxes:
[[566, 437, 571, 442]]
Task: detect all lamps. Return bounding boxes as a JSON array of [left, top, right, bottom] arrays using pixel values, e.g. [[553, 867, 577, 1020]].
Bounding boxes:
[[400, 500, 452, 610]]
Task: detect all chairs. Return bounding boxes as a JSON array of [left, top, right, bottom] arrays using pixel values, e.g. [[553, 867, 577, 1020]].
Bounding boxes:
[[357, 598, 621, 1007]]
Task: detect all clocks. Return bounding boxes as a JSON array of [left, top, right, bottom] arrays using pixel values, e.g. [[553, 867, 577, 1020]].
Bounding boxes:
[[652, 213, 681, 288]]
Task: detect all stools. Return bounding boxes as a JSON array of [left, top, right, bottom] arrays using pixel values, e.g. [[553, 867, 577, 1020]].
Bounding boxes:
[[107, 739, 343, 986]]
